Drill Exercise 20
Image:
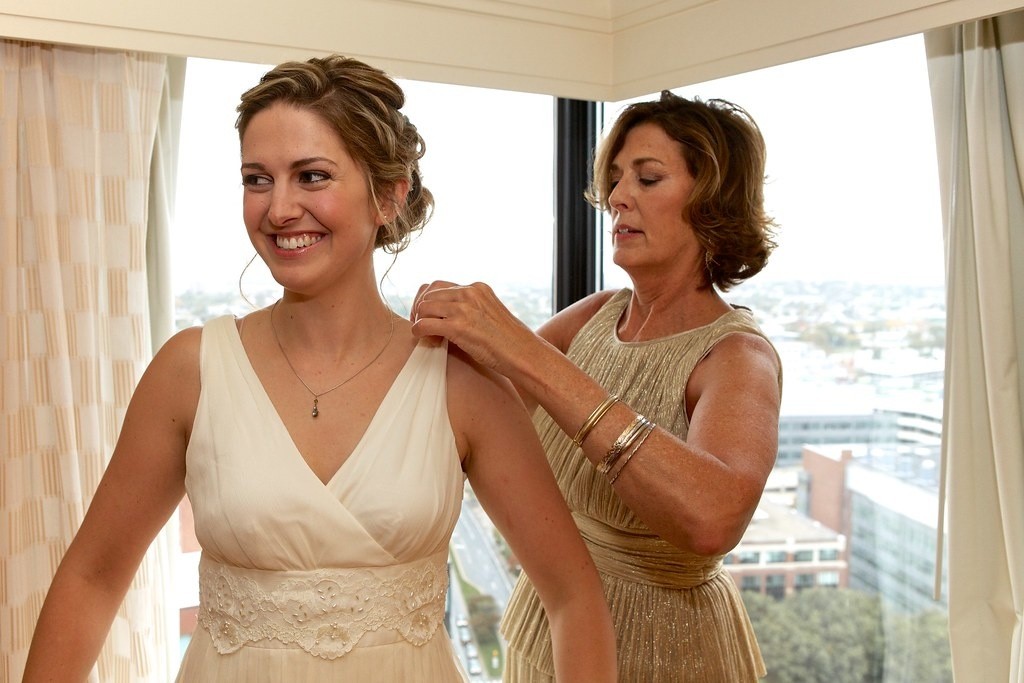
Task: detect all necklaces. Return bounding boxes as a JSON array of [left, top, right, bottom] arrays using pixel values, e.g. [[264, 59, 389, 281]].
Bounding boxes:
[[270, 298, 393, 417]]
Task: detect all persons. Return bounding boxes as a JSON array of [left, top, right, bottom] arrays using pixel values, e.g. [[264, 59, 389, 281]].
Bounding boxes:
[[22, 54, 619, 683], [411, 89, 783, 683]]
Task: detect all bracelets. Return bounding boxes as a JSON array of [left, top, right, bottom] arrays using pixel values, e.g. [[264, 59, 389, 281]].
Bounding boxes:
[[572, 394, 621, 447], [595, 413, 657, 485]]
